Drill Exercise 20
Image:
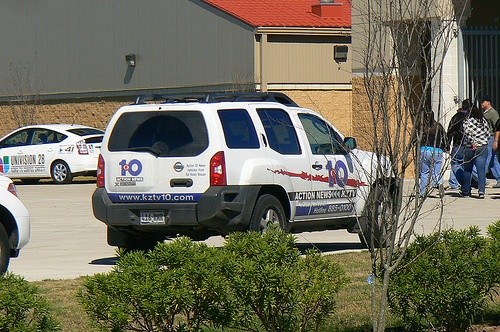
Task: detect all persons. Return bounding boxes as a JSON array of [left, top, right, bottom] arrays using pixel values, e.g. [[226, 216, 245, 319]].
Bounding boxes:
[[419, 110, 448, 197], [448, 96, 500, 198]]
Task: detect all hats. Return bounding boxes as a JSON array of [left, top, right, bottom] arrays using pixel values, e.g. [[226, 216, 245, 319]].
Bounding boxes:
[[478, 95, 492, 103]]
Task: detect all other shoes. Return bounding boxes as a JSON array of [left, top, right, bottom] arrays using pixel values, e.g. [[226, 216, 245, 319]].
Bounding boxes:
[[439, 183, 444, 198], [492, 182, 500, 188], [458, 191, 469, 198], [479, 194, 484, 198], [419, 197, 423, 201], [446, 186, 459, 191]]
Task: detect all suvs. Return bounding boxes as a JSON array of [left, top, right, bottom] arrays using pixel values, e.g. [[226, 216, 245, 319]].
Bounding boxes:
[[90, 90, 400, 255]]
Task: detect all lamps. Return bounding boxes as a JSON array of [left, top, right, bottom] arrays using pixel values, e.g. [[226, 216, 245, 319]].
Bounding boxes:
[[334, 45, 348, 59], [125, 53, 137, 68]]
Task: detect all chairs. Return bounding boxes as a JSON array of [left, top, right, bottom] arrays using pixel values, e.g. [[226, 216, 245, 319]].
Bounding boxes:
[[38, 134, 47, 144]]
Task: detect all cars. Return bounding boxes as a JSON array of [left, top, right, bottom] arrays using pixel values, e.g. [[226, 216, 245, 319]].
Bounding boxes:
[[0, 123, 106, 184], [0, 175, 31, 275]]
